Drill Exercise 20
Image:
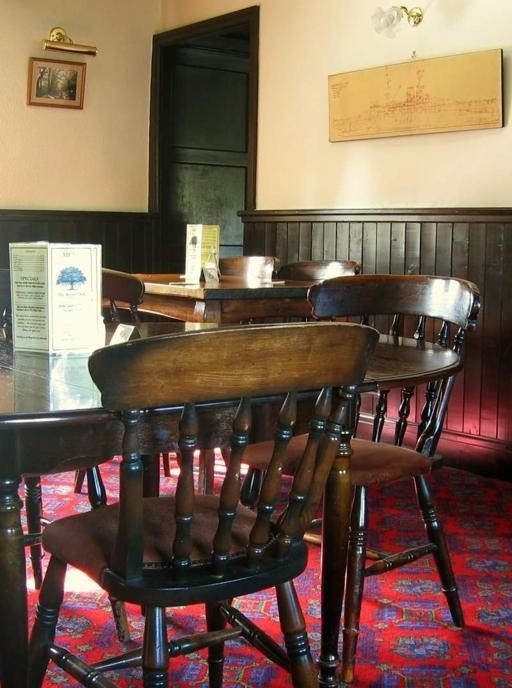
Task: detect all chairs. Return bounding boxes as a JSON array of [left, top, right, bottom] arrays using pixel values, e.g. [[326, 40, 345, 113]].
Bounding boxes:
[[276, 256, 365, 286], [17, 316, 382, 686], [218, 254, 280, 281], [210, 272, 480, 683], [25, 260, 146, 324]]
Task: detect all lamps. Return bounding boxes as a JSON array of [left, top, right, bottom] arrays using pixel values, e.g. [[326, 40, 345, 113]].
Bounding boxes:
[[373, 5, 423, 35]]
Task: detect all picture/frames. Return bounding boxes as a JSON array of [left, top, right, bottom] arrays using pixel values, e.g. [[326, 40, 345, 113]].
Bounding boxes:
[[27, 55, 87, 110]]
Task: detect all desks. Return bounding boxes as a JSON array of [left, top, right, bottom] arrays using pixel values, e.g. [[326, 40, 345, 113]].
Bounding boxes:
[[0, 330, 462, 688], [133, 269, 336, 327]]
